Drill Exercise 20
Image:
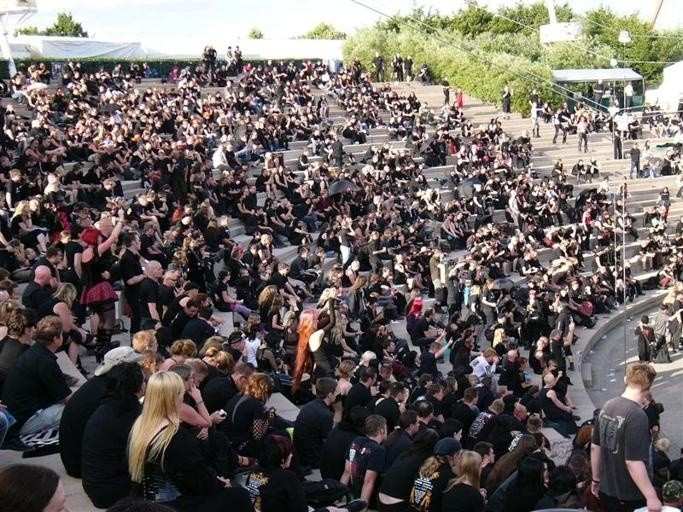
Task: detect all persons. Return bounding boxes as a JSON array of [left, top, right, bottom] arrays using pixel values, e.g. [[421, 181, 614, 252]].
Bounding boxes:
[[1, 41, 682, 512]]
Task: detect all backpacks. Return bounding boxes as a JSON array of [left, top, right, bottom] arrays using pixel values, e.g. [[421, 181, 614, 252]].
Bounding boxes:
[[303, 479, 351, 508]]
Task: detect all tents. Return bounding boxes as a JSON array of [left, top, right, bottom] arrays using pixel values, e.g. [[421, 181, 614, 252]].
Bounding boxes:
[[1, 35, 344, 72]]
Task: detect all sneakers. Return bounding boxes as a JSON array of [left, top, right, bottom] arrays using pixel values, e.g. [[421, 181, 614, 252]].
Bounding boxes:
[[339, 499, 367, 512]]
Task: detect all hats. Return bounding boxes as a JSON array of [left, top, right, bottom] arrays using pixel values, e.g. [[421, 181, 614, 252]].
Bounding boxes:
[[434, 437, 461, 455]]
